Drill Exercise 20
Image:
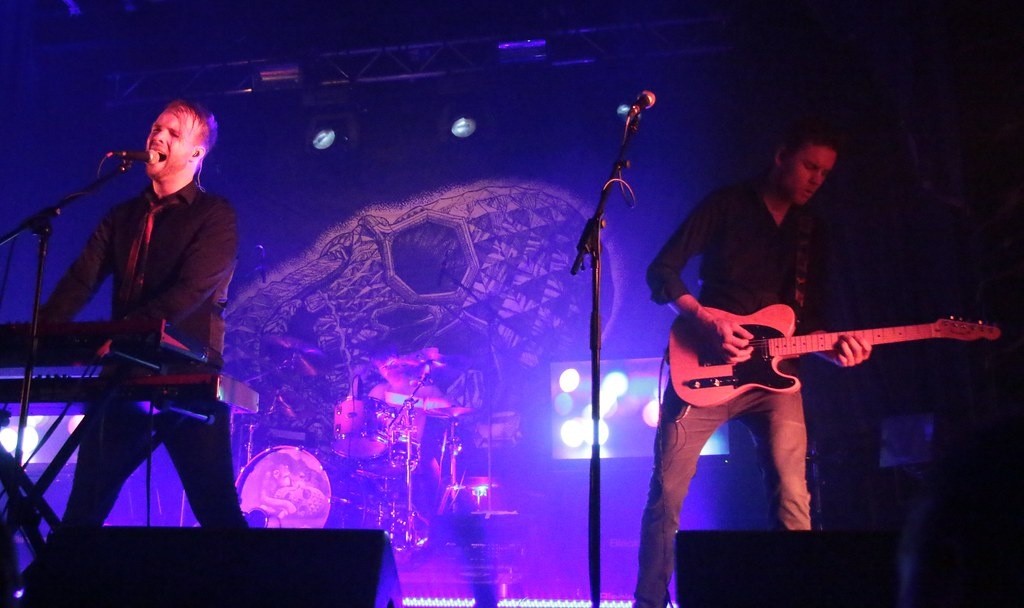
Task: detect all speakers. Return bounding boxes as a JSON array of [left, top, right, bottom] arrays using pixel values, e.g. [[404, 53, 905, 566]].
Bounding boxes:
[[22, 526, 403, 608], [675, 530, 900, 608]]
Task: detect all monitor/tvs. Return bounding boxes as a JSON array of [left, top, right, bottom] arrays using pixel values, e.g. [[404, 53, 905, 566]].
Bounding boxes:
[[542, 353, 738, 475]]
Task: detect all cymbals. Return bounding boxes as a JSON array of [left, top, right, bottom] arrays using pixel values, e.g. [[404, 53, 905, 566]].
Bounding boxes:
[[424, 407, 478, 417]]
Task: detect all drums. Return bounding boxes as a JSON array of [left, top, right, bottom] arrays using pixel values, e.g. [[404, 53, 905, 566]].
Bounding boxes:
[[233, 443, 364, 528], [334, 395, 419, 471]]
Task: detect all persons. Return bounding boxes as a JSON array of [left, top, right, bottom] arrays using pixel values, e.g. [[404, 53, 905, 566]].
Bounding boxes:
[[36, 97, 246, 529], [634, 125, 873, 608]]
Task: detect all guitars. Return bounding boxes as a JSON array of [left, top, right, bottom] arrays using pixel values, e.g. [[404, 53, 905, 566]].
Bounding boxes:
[[668, 304, 1001, 407]]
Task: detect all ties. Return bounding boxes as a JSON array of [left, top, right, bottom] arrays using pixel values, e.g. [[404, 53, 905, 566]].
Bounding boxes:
[[108, 198, 179, 321]]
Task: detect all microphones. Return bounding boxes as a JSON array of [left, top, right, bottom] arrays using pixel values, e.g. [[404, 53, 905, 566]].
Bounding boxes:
[[114, 149, 160, 166], [627, 89, 656, 118]]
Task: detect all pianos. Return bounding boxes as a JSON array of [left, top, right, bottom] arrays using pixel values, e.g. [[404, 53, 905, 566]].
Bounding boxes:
[[0, 321, 259, 608]]
